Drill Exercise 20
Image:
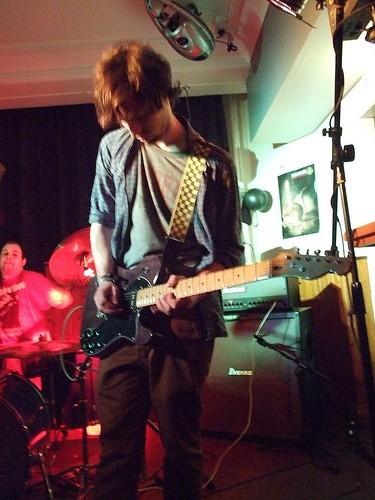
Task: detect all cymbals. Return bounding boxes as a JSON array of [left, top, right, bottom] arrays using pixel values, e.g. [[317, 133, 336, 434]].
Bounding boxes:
[[49, 228, 96, 286], [0, 335, 81, 358]]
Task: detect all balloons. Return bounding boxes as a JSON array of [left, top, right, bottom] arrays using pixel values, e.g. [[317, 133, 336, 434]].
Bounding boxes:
[[244, 188, 268, 212]]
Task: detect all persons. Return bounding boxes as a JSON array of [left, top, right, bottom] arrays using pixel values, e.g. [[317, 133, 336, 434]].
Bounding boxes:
[[89, 40, 245, 500], [0, 242, 74, 391]]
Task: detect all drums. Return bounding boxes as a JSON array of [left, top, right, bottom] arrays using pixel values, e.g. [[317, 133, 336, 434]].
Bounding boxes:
[[0, 371, 50, 466]]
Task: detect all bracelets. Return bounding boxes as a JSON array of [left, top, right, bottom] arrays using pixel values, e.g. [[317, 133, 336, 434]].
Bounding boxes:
[[95, 274, 115, 286]]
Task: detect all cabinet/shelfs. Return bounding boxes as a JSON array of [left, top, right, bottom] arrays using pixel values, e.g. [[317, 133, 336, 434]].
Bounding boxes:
[[197, 307, 320, 439]]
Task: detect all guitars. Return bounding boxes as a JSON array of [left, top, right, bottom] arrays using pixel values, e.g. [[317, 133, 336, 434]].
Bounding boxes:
[[80, 249, 353, 358]]
